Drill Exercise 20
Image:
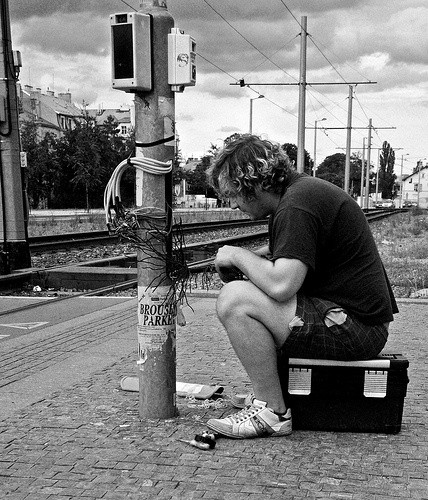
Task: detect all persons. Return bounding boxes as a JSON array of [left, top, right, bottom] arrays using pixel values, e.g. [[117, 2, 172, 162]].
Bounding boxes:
[[205, 133, 400, 439]]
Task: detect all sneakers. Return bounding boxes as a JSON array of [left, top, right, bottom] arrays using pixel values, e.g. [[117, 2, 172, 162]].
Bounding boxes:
[[208, 400, 291, 438], [230, 392, 254, 408]]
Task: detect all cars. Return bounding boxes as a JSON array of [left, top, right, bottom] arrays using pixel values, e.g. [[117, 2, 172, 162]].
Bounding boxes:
[[373, 199, 382, 211], [382, 198, 394, 212], [402, 200, 411, 208], [412, 201, 418, 207]]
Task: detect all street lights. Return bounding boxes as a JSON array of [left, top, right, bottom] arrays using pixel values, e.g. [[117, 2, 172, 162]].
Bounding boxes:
[[250, 94, 266, 135], [357, 133, 374, 207], [399, 153, 409, 210], [418, 158, 426, 215], [313, 117, 330, 177]]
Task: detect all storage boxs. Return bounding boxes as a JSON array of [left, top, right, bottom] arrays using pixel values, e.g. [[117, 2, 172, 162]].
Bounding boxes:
[[278, 351, 410, 435]]
